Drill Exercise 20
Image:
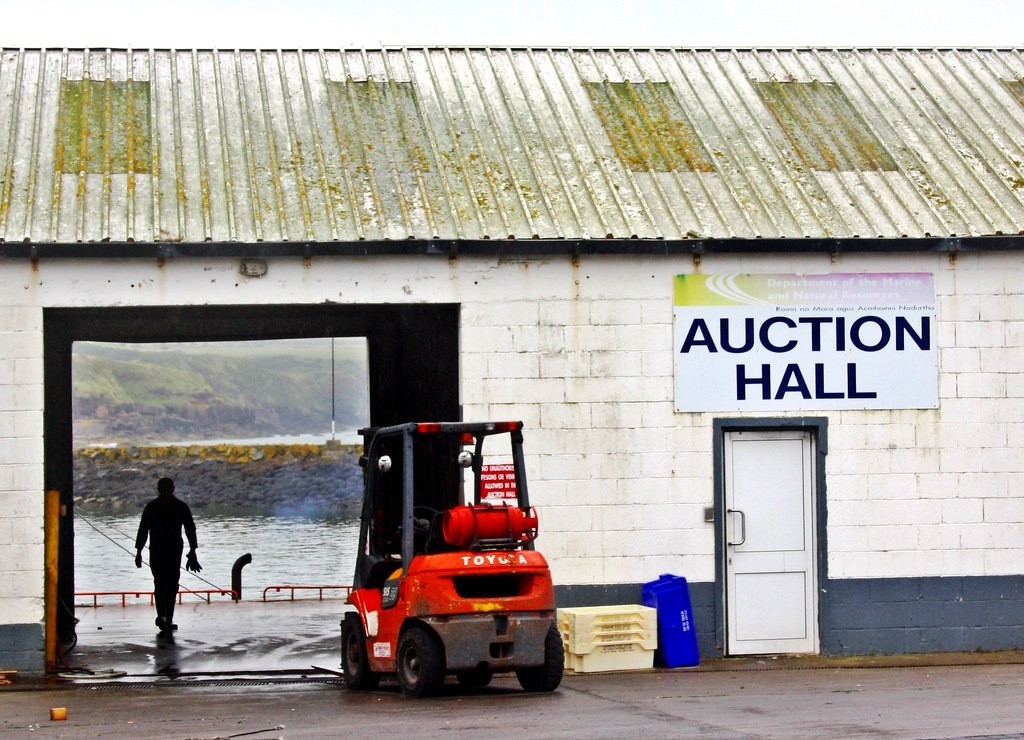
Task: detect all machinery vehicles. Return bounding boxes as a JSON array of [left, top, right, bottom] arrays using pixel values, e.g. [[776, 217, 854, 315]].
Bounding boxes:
[[340, 417, 566, 700]]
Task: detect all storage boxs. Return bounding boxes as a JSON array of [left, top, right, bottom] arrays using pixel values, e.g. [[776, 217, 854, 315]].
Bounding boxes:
[[641, 573, 700, 669], [556, 604, 658, 673]]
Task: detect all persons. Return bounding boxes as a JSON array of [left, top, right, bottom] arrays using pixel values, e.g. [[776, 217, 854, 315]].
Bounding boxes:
[[135, 478, 202, 630]]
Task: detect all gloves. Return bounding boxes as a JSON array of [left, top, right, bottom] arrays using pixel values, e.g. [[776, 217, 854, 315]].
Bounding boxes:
[[186, 554, 202, 573]]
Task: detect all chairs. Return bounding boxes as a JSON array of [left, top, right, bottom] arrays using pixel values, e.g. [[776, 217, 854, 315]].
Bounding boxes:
[[424, 511, 466, 555]]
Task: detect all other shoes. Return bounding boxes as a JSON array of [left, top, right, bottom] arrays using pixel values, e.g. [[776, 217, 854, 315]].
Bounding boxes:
[[168, 623, 178, 630], [156, 616, 169, 630]]
